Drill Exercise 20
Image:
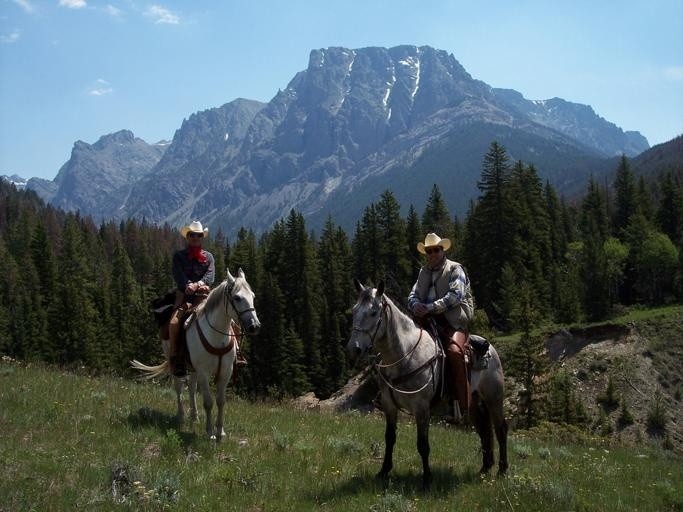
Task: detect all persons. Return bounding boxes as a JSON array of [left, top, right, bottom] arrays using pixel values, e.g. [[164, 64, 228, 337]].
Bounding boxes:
[[167, 220, 248, 379], [404, 232, 475, 427]]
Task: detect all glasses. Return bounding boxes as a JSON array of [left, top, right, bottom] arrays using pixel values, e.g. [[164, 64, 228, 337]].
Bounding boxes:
[[425, 247, 442, 254], [189, 232, 204, 238]]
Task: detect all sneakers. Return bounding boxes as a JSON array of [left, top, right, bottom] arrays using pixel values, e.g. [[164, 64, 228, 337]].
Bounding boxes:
[[170, 361, 186, 376], [445, 409, 472, 425], [235, 351, 248, 366]]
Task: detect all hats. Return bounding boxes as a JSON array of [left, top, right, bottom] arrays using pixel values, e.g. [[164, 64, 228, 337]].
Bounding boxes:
[[180, 220, 208, 239], [416, 233, 452, 255]]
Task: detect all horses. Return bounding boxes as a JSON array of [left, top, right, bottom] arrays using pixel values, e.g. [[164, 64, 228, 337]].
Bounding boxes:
[[347, 278, 508, 486], [130, 268, 262, 444]]
